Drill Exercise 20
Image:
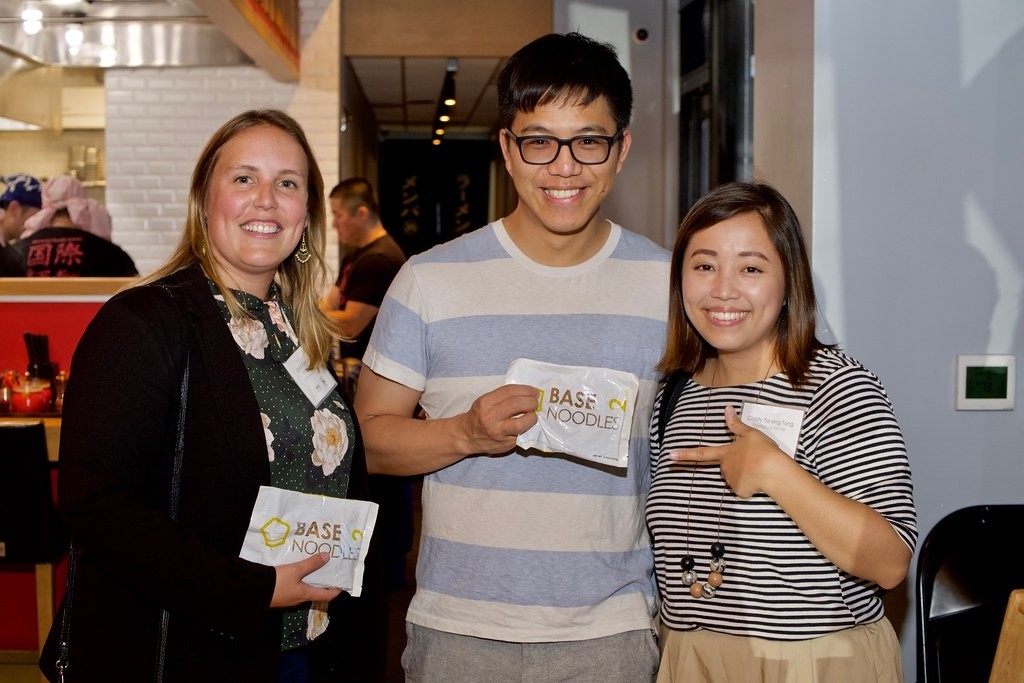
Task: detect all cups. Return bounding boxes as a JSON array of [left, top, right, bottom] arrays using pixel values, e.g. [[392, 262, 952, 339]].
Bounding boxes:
[[27, 361, 58, 401]]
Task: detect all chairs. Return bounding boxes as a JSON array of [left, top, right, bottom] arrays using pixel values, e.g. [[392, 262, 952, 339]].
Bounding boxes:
[[0, 424, 67, 683], [916, 504, 1024, 683]]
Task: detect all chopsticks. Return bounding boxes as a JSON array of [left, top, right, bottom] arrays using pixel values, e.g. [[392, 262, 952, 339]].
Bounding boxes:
[[23, 333, 49, 363]]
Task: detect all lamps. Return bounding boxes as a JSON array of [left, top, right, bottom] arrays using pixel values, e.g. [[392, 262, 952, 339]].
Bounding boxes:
[[432, 71, 456, 145]]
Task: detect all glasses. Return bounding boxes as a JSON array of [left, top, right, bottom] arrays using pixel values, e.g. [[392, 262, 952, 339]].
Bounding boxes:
[[504, 126, 624, 165]]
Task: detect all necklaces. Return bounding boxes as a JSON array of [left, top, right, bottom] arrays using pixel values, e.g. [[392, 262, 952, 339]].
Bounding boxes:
[[680, 355, 777, 599]]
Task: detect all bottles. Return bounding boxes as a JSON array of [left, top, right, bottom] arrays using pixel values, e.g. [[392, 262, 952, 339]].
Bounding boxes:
[[11, 382, 52, 414], [54, 370, 69, 413]]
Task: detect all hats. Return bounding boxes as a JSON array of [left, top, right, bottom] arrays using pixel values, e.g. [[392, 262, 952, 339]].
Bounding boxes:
[[0, 175, 42, 209]]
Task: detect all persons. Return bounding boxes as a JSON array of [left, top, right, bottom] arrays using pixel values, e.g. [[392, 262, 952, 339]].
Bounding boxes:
[[351, 31, 674, 683], [314, 177, 420, 593], [644, 182, 919, 683], [50, 109, 368, 683], [0, 174, 138, 277]]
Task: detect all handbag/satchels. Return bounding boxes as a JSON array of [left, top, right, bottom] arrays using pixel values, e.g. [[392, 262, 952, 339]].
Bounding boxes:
[[38, 571, 171, 683]]
